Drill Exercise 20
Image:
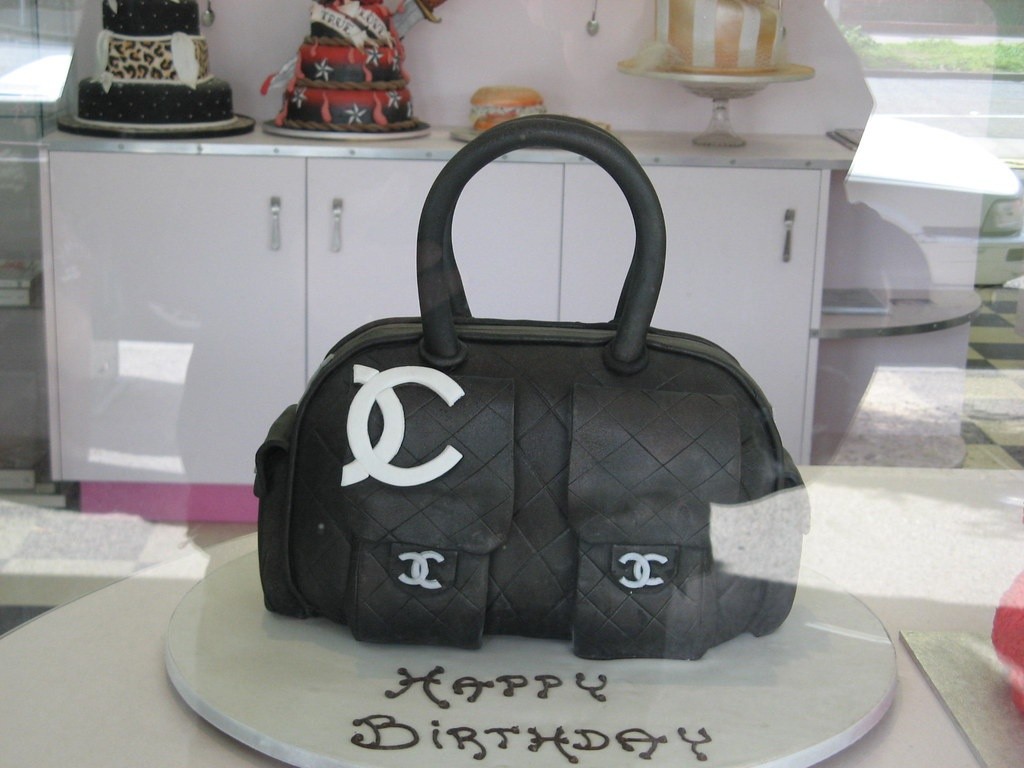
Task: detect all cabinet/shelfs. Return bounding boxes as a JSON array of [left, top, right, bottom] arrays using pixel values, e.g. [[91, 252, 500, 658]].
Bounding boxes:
[[36, 118, 856, 522]]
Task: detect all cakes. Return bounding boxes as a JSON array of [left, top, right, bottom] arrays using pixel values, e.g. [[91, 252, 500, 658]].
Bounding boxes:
[[650, 0, 786, 72], [78, 0, 233, 124], [470, 86, 545, 133], [274, 0, 420, 130]]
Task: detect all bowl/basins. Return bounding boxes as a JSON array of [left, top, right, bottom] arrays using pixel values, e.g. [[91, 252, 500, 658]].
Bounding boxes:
[[469, 86, 547, 133]]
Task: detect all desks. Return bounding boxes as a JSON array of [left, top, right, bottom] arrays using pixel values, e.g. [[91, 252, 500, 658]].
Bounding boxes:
[[0, 469, 1024, 768]]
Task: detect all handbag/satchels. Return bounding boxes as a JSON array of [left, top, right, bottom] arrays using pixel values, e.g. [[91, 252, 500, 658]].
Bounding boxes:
[[253, 114, 812, 661]]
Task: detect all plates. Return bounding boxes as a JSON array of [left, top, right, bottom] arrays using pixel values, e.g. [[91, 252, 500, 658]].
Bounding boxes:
[[451, 120, 611, 142], [261, 118, 430, 140], [54, 113, 256, 137]]
